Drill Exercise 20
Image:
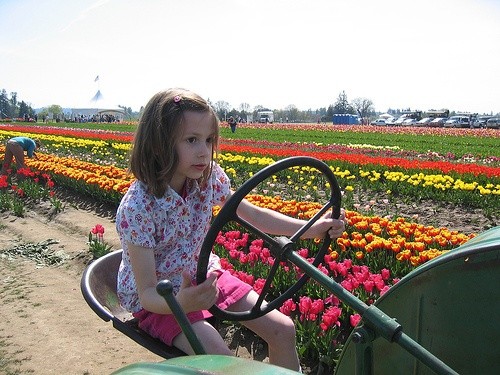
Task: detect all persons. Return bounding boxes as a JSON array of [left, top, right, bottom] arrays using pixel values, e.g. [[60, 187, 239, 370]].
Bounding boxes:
[[227, 114, 247, 133], [116, 86, 347, 373], [278, 115, 289, 123], [21, 110, 120, 123], [2, 137, 41, 170]]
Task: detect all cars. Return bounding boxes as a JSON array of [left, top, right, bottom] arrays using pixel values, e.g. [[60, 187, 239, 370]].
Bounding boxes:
[[369, 112, 500, 129]]
